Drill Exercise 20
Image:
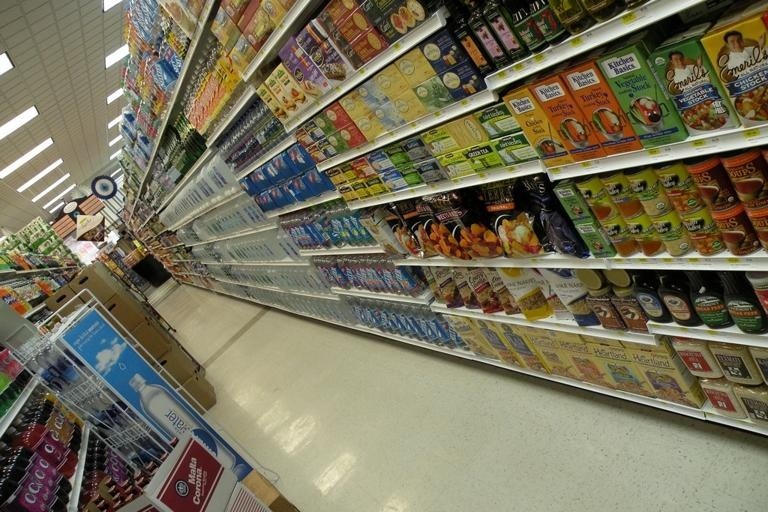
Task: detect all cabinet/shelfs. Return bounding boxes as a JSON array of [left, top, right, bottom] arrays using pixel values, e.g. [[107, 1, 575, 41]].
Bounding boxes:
[[203, 0, 705, 431], [136, 100, 359, 333], [0, 247, 78, 331], [125, 199, 215, 295], [0, 338, 171, 512], [441, 0, 768, 443], [177, 21, 474, 370]]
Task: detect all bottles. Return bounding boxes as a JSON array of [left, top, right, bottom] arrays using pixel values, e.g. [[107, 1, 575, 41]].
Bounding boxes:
[[0, 389, 13, 418], [0, 372, 22, 406], [749, 347, 768, 385], [0, 441, 72, 494], [25, 402, 81, 443], [632, 276, 672, 323], [6, 427, 75, 479], [483, 0, 532, 63], [527, 0, 570, 47], [18, 414, 78, 457], [575, 269, 605, 290], [610, 287, 649, 333], [121, 0, 289, 194], [0, 346, 31, 386], [602, 269, 638, 287], [0, 252, 77, 270], [586, 285, 628, 334], [658, 277, 704, 327], [746, 272, 768, 314], [280, 199, 377, 250], [547, 0, 593, 35], [343, 296, 469, 353], [732, 383, 768, 425], [79, 438, 179, 512], [130, 374, 237, 472], [684, 270, 735, 329], [29, 395, 82, 436], [312, 253, 428, 297], [0, 477, 54, 512], [0, 466, 67, 512], [465, 4, 512, 70], [717, 271, 768, 334], [81, 388, 139, 438], [582, 0, 625, 23], [701, 378, 747, 419], [0, 274, 70, 317], [33, 388, 83, 427], [451, 20, 499, 76], [22, 407, 80, 451], [670, 337, 724, 378], [0, 456, 70, 503], [13, 419, 77, 467], [30, 304, 88, 392], [506, 0, 550, 54], [708, 343, 764, 386]]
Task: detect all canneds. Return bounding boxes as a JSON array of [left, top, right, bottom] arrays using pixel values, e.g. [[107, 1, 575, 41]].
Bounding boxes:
[[687, 158, 737, 212], [575, 176, 619, 222], [722, 151, 768, 208], [600, 217, 641, 257], [678, 206, 724, 254], [623, 168, 673, 219], [710, 204, 763, 256], [624, 215, 666, 255], [745, 207, 768, 252], [655, 161, 704, 215], [648, 211, 693, 257], [599, 173, 645, 221]]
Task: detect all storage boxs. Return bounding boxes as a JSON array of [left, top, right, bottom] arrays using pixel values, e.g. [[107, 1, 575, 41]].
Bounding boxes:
[[123, 316, 174, 367], [69, 260, 119, 309], [84, 434, 237, 512], [152, 344, 196, 390], [95, 289, 145, 340], [45, 285, 91, 322], [174, 371, 217, 415]]
